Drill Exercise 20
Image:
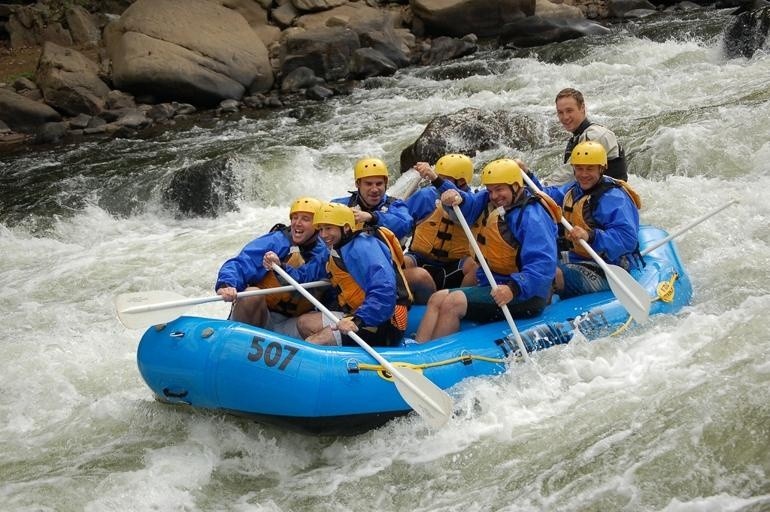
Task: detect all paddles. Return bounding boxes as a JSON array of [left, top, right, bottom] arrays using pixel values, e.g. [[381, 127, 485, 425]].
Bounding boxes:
[[116, 280, 332, 328], [272, 261, 453, 426], [521, 169, 651, 324]]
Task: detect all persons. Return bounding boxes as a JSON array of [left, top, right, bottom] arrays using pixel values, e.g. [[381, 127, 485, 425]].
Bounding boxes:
[[263, 202, 397, 348], [330, 158, 414, 246], [404, 158, 557, 344], [556, 89, 628, 185], [215, 196, 355, 346], [513, 141, 647, 304], [391, 154, 480, 304]]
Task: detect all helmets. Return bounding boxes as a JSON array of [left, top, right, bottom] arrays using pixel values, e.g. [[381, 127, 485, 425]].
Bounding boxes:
[[481, 158, 524, 188], [289, 197, 323, 219], [313, 202, 355, 231], [570, 141, 607, 169], [435, 154, 473, 185], [354, 157, 388, 191]]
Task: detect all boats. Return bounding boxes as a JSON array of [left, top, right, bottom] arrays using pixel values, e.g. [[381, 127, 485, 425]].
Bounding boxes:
[[138, 225, 692, 421]]
